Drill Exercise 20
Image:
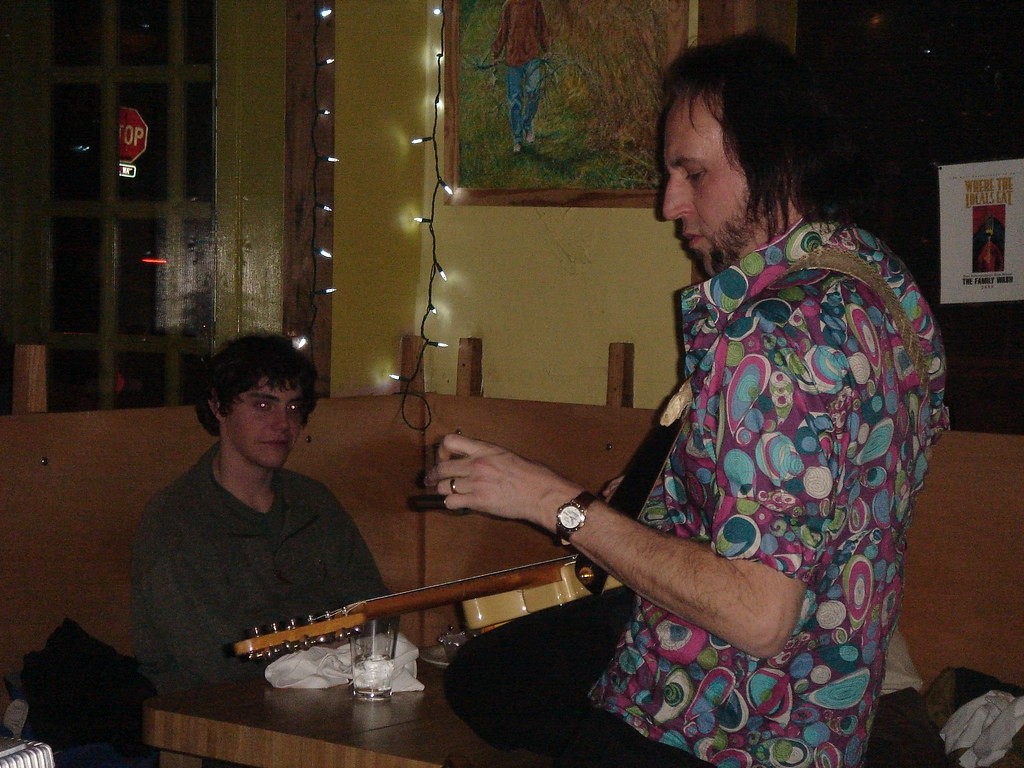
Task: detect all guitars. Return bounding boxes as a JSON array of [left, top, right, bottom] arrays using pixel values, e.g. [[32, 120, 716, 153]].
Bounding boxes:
[[229, 550, 625, 670]]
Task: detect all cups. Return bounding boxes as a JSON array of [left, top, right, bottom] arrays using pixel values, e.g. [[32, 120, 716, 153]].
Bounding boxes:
[[350, 614, 401, 703]]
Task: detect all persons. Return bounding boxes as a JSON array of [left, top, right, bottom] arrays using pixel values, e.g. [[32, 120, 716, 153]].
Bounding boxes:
[[423, 29, 948, 768], [129, 330, 400, 697]]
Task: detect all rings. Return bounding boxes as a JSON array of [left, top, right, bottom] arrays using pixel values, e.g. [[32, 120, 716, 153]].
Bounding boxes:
[[450, 478, 457, 493]]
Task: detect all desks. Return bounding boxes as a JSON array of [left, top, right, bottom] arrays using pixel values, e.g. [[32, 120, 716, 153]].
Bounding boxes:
[[141, 646, 559, 768]]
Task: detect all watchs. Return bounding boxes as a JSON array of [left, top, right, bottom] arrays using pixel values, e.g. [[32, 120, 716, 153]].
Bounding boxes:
[[555, 490, 599, 545]]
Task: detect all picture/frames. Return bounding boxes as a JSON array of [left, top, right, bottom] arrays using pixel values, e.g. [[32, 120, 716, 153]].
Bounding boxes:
[[440, 0, 691, 209]]
